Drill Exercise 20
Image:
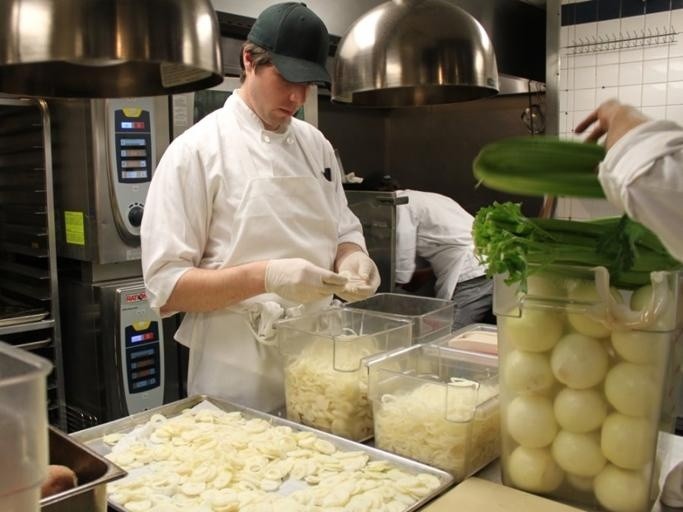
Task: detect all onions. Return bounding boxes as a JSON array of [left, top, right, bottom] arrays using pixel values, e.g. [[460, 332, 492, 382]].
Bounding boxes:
[[501, 271, 677, 512]]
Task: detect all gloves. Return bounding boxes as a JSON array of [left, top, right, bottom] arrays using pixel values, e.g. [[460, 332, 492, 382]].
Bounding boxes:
[[262, 256, 347, 305], [333, 249, 384, 306]]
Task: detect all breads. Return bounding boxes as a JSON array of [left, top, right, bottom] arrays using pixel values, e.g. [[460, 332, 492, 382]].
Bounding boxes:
[[40, 465, 78, 497]]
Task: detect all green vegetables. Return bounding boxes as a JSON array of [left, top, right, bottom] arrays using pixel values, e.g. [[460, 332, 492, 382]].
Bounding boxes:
[[473, 201, 680, 294]]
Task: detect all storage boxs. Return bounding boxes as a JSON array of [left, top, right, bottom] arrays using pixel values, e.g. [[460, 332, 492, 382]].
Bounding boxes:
[[492, 261, 683, 512], [0, 341, 54, 512]]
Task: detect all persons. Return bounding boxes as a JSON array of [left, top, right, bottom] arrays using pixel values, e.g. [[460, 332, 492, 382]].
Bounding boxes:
[[139, 1, 381, 415], [360, 174, 494, 332], [574, 98, 683, 266]]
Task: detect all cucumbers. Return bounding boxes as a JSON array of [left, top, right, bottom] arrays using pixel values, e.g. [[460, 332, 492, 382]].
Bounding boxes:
[[473, 138, 606, 199]]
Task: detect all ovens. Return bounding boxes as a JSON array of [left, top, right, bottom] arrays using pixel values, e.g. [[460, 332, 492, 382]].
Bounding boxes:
[[52, 74, 320, 421]]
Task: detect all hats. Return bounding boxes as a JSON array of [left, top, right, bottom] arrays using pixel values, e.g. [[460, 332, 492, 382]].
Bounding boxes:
[[244, 1, 333, 87]]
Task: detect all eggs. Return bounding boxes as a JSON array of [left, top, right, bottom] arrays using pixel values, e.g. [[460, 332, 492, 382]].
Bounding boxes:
[[501, 271, 677, 512]]
